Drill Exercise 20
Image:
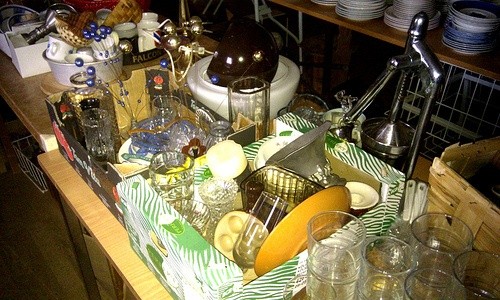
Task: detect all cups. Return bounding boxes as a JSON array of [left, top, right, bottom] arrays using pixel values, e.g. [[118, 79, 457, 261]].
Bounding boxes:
[[137, 13, 161, 52], [81, 109, 111, 157], [282, 211, 500, 300], [233, 191, 288, 269], [228, 76, 270, 142], [149, 96, 238, 241], [45, 34, 115, 64]]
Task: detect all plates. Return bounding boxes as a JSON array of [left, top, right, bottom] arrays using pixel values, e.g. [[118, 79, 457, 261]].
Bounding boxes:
[[311, 0, 447, 32], [255, 185, 352, 277], [345, 182, 379, 210]]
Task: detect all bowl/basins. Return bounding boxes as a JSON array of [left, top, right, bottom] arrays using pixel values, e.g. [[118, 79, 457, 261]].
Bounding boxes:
[[42, 50, 123, 86], [287, 94, 329, 128], [114, 22, 136, 38], [440, 0, 500, 55]]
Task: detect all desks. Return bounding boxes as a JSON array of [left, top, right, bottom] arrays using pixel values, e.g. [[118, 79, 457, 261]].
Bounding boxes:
[[0, 0, 500, 300]]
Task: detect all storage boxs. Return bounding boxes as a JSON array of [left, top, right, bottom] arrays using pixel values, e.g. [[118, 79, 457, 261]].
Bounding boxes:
[[5, 31, 61, 79], [43, 65, 255, 229], [427, 134, 500, 254], [117, 112, 406, 300]]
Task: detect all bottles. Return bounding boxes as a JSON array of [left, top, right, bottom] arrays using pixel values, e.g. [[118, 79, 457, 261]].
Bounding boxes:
[[68, 72, 119, 136]]
